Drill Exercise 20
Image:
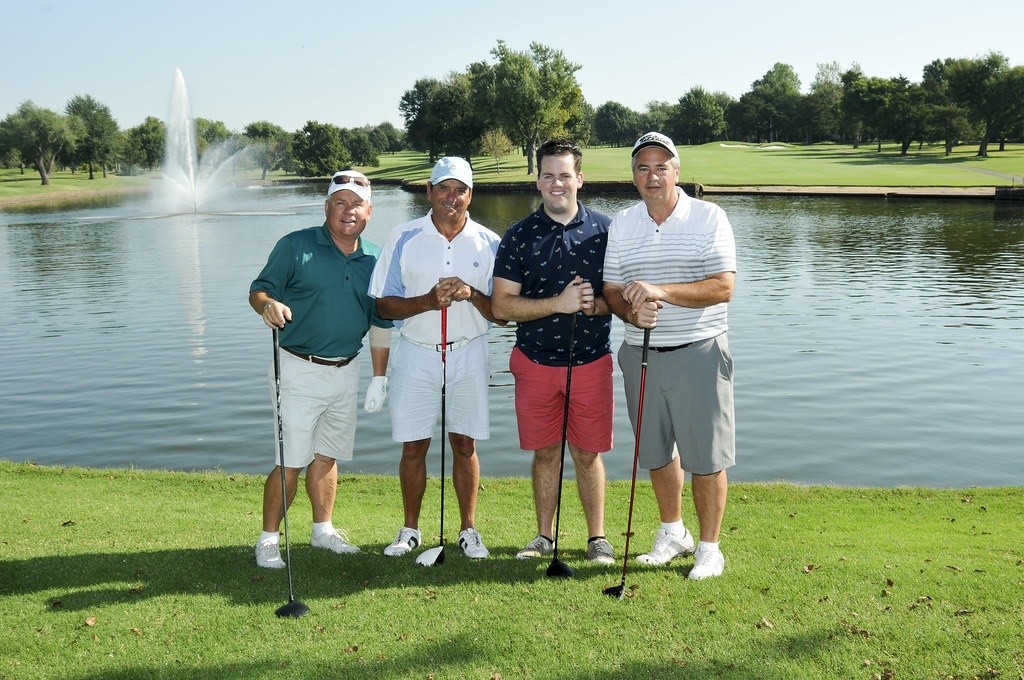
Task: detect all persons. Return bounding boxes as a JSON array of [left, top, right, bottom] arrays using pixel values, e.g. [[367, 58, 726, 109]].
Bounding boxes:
[[603, 132, 735, 580], [368, 157, 508, 559], [490, 138, 614, 563], [249, 171, 393, 567]]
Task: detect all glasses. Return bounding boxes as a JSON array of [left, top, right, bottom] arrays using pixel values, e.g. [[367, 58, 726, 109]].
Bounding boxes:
[[328, 175, 370, 189]]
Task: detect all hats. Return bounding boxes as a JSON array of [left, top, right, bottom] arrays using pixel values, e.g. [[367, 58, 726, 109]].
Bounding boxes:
[[631, 131, 679, 160], [327, 170, 371, 203], [429, 156, 473, 190]]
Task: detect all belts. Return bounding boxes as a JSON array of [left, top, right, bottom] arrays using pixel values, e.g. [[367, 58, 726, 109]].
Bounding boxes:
[[401, 333, 471, 352], [281, 346, 361, 368], [639, 342, 692, 353]]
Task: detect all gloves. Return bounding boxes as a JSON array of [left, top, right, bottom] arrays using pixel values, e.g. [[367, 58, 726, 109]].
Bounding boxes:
[[364, 376, 388, 413]]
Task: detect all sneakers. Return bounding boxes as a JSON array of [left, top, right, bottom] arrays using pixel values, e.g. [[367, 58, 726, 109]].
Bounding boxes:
[[254, 540, 287, 569], [516, 534, 556, 559], [458, 528, 490, 559], [309, 528, 361, 555], [587, 538, 615, 564], [686, 543, 724, 580], [636, 528, 695, 567], [383, 527, 421, 556]]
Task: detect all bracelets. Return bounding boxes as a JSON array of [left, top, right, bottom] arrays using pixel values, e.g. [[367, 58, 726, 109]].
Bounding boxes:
[[468, 287, 478, 302]]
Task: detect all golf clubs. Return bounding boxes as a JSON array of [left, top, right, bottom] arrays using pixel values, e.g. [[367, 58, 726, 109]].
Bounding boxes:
[[413, 306, 448, 567], [601, 298, 654, 603], [272, 324, 313, 619], [545, 283, 580, 580]]
